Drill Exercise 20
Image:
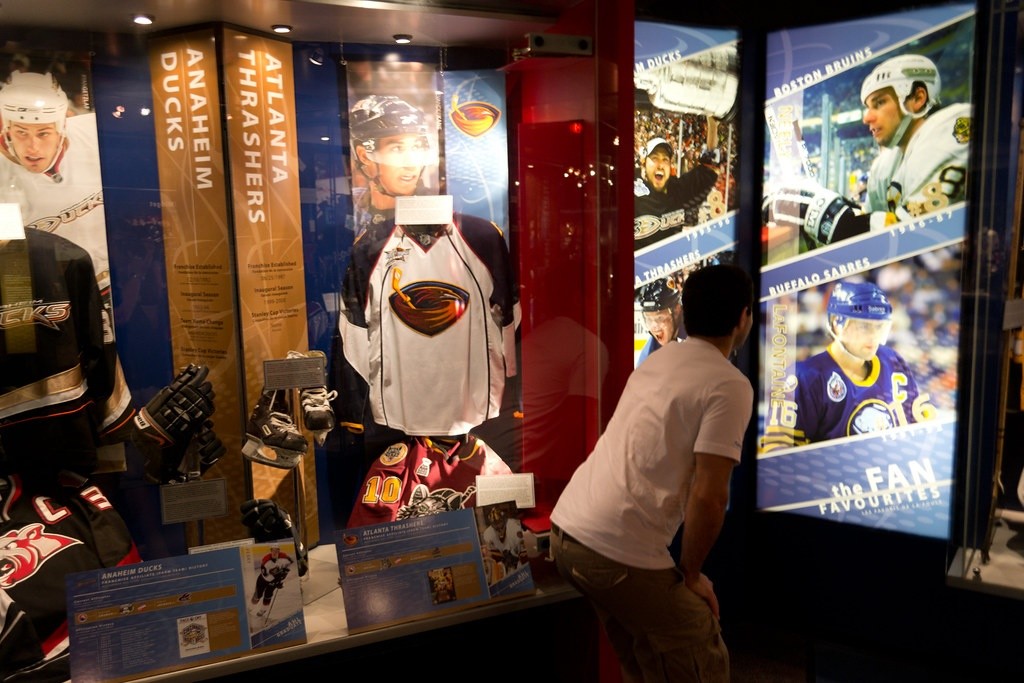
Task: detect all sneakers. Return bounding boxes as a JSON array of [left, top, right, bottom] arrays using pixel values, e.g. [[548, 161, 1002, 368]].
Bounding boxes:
[[242, 385, 308, 469], [286, 350, 338, 447], [257, 608, 266, 616]]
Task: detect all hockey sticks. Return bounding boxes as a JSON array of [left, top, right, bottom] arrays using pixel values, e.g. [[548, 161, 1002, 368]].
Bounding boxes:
[[264, 588, 279, 628]]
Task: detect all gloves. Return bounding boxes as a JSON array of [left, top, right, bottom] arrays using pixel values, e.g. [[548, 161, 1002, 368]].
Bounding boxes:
[[146, 419, 227, 484], [240, 499, 306, 560], [129, 364, 216, 466], [274, 567, 290, 581], [761, 186, 850, 246]]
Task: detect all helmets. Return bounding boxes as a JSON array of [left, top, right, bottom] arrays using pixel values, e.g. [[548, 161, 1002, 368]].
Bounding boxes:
[[349, 95, 430, 164], [861, 54, 942, 119], [270, 543, 281, 550], [0, 70, 68, 134], [488, 507, 506, 524], [827, 278, 892, 330], [638, 277, 681, 312]]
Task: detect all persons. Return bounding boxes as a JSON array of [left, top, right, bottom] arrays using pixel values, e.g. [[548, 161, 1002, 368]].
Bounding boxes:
[[637, 273, 687, 368], [634, 114, 725, 244], [247, 542, 295, 619], [346, 93, 445, 244], [762, 52, 971, 247], [0, 70, 118, 404], [548, 253, 755, 683], [482, 506, 528, 576], [758, 277, 936, 455]]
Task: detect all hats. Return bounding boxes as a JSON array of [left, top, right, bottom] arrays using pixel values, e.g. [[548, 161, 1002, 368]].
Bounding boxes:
[[639, 138, 674, 158]]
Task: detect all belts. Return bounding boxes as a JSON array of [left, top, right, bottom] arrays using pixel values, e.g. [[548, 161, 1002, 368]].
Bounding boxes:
[[551, 522, 577, 544]]
